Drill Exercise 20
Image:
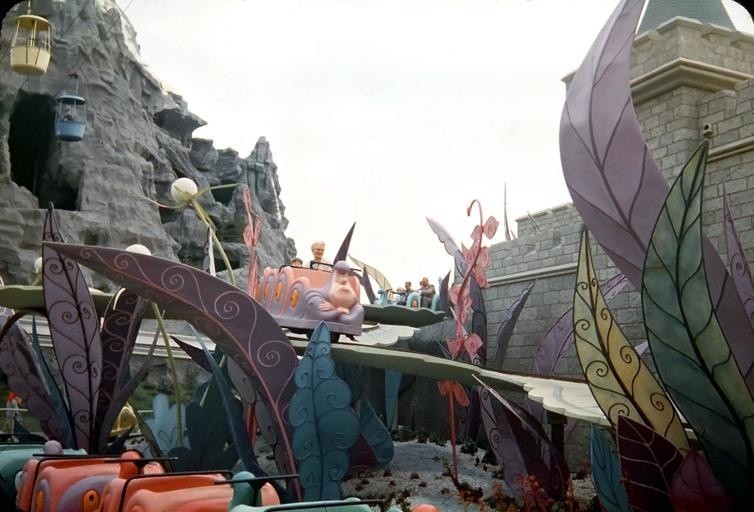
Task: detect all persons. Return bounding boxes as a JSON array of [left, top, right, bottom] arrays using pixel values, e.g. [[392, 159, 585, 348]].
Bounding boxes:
[[396, 282, 418, 306], [417, 277, 437, 307], [291, 258, 303, 267], [311, 240, 333, 264]]
